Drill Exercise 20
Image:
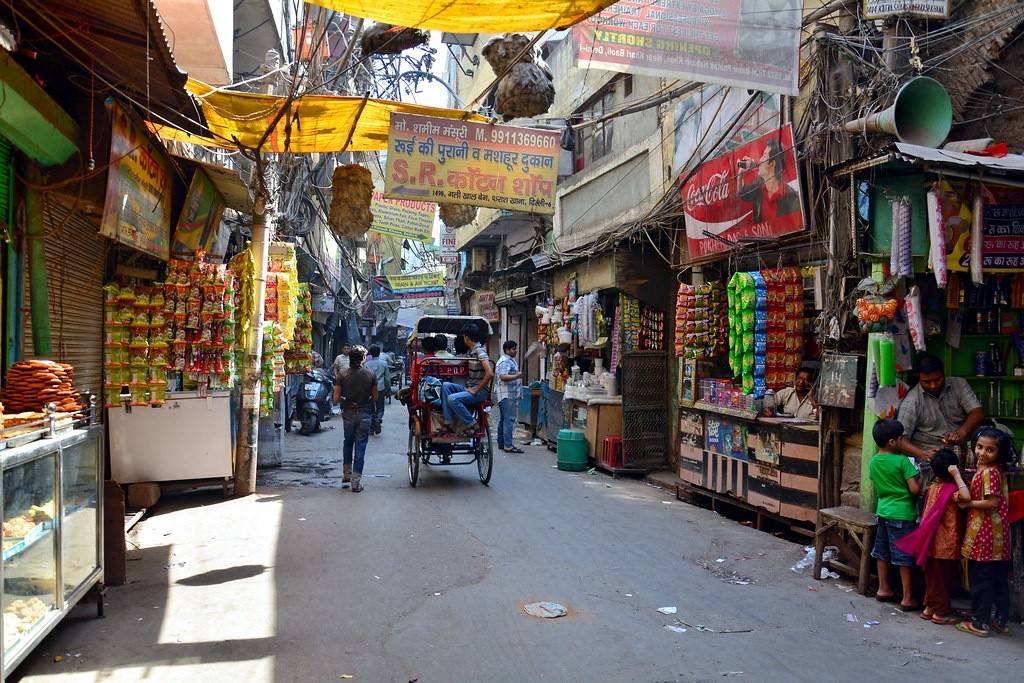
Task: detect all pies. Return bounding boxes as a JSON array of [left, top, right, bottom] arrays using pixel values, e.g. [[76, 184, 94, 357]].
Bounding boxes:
[[0, 360, 87, 411]]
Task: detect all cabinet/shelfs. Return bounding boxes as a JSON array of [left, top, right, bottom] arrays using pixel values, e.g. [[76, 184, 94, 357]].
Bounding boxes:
[[904, 330, 1024, 453]]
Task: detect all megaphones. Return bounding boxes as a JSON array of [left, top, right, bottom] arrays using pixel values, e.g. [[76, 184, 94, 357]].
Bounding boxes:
[[844, 76, 953, 150]]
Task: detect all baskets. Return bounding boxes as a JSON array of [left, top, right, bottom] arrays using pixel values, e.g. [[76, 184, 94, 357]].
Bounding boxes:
[[856, 296, 900, 334]]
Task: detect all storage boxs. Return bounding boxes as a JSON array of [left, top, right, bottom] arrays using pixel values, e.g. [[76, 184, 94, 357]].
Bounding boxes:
[[603, 435, 624, 467]]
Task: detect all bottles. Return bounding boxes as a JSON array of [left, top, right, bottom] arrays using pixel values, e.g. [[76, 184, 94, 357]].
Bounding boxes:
[[965, 441, 976, 469], [988, 342, 1003, 375], [944, 270, 959, 309], [737, 159, 758, 170], [953, 445, 965, 472], [699, 377, 778, 418], [976, 311, 994, 334], [556, 362, 590, 392], [976, 378, 1024, 418]]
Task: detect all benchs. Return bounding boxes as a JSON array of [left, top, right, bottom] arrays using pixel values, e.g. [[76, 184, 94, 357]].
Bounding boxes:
[[410, 357, 492, 407]]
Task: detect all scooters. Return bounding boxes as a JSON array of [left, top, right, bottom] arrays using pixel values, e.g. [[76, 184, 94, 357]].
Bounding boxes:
[[292, 368, 334, 435]]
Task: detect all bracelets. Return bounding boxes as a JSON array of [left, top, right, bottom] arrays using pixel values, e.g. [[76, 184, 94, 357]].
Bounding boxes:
[[958, 485, 966, 489]]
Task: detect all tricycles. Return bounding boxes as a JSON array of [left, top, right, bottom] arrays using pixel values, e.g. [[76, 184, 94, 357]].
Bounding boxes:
[[392, 314, 497, 488]]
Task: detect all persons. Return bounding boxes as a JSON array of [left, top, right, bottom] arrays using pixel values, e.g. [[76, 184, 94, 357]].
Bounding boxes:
[[776, 366, 818, 419], [311, 344, 323, 368], [333, 344, 378, 492], [364, 345, 391, 435], [957, 428, 1012, 639], [434, 334, 455, 363], [895, 448, 971, 624], [897, 354, 985, 462], [435, 324, 493, 436], [379, 347, 395, 366], [869, 418, 924, 611], [447, 336, 470, 363], [737, 138, 801, 224], [410, 337, 445, 400], [334, 344, 350, 411], [495, 341, 524, 453]]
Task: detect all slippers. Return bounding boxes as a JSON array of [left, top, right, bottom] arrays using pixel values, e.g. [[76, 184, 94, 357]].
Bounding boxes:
[[342, 479, 351, 487], [505, 446, 524, 453], [498, 445, 504, 449], [921, 613, 932, 619], [932, 614, 961, 624], [956, 621, 989, 637], [352, 484, 363, 492], [991, 619, 1012, 635], [901, 599, 926, 612], [876, 592, 903, 602]]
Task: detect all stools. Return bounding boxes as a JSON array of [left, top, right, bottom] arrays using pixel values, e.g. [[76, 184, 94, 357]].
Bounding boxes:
[[812, 505, 881, 596]]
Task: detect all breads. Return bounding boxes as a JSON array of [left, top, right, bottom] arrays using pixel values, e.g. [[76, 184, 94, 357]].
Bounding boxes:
[[1, 500, 56, 652]]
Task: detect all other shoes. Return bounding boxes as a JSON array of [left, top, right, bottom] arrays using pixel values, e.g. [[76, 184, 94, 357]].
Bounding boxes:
[[369, 431, 374, 435], [462, 426, 481, 435], [435, 425, 456, 434], [375, 420, 381, 434]]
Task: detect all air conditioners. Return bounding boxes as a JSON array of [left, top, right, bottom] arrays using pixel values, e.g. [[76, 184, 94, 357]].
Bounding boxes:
[[471, 247, 488, 272]]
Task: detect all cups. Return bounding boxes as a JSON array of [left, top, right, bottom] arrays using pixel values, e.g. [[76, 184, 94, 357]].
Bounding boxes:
[[973, 351, 987, 377], [594, 358, 603, 376]]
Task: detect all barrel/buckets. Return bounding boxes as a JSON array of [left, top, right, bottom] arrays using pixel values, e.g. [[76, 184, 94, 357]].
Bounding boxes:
[[557, 429, 589, 472]]
